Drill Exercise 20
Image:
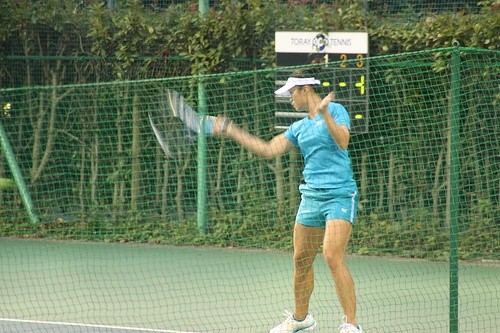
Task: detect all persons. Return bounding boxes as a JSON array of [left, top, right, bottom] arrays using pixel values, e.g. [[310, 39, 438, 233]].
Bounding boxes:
[[199, 66, 362, 333]]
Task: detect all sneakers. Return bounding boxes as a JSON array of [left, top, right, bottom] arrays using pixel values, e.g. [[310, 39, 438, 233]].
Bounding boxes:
[[337, 315, 365, 333], [270, 310, 316, 333]]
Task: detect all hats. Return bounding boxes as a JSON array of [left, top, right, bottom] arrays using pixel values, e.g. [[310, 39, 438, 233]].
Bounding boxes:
[[274, 77, 320, 94]]
